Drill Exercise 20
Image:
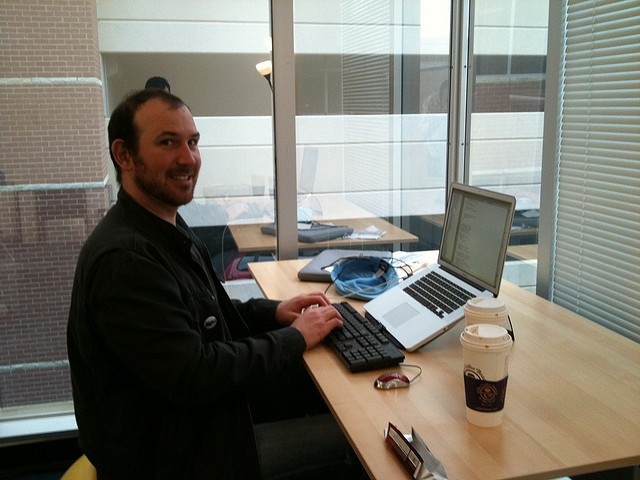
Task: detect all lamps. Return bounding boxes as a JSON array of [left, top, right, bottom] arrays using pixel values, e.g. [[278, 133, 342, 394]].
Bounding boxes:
[[255, 60, 273, 92]]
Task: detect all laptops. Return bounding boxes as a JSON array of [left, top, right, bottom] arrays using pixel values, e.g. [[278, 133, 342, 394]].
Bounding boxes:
[[297, 146, 320, 196], [362, 180, 518, 354], [259, 214, 355, 244], [295, 247, 393, 284]]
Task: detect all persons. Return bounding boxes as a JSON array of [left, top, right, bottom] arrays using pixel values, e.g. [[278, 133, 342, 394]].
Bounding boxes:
[[144, 75, 171, 93], [67, 85, 344, 478]]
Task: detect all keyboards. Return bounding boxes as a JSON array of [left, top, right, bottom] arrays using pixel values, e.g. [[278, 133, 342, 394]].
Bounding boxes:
[[313, 301, 407, 373], [241, 201, 262, 221]]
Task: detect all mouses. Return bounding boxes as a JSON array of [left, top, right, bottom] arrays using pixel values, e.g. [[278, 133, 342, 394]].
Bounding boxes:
[[373, 371, 412, 391]]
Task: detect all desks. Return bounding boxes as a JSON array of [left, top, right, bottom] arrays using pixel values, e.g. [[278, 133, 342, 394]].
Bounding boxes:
[[246, 250, 640, 480], [228, 217, 420, 253]]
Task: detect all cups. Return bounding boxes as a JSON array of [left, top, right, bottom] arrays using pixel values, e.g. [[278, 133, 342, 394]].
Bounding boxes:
[[465, 297, 509, 329], [252, 168, 267, 195]]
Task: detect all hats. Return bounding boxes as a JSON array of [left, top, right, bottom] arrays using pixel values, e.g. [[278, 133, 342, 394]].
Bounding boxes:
[[331, 253, 399, 301]]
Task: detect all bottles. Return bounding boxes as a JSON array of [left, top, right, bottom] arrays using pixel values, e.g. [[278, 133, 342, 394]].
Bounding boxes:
[[461, 324, 514, 427]]
